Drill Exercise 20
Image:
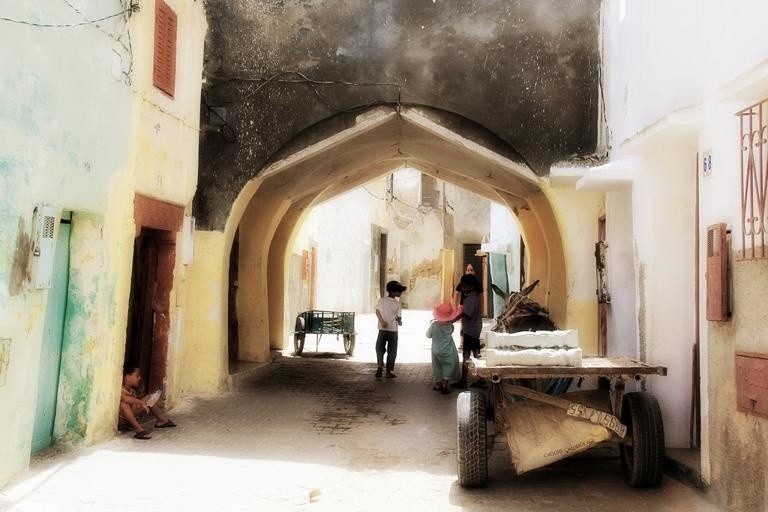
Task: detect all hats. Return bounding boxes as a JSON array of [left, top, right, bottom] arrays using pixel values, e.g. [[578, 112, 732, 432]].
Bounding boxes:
[[432, 302, 461, 323], [386, 279, 407, 293]]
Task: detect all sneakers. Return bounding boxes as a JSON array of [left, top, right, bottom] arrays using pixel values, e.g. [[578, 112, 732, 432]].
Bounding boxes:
[[470, 380, 487, 389], [450, 380, 468, 390], [433, 383, 451, 394], [374, 370, 396, 379]]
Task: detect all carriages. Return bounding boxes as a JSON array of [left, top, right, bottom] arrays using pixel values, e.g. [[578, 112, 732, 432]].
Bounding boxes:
[[457, 279, 668, 490]]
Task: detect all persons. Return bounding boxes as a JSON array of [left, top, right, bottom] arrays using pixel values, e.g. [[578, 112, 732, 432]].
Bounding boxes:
[[447, 273, 490, 390], [425, 302, 462, 396], [116, 360, 178, 440], [452, 263, 486, 350], [374, 279, 407, 379]]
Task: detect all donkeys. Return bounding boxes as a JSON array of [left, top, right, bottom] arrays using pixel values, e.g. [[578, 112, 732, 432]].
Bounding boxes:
[[489, 279, 574, 401]]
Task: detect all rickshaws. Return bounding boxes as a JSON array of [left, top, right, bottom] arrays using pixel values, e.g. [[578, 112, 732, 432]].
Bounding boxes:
[[293, 310, 356, 355]]
